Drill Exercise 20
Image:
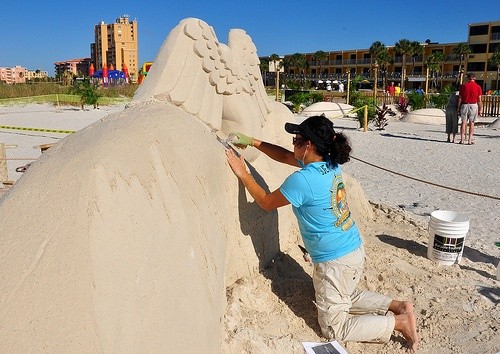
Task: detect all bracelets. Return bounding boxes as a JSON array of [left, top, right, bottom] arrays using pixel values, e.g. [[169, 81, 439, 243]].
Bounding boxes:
[[251, 137, 254, 147]]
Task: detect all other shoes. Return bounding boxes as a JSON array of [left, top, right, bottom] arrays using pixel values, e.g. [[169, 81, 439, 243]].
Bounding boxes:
[[447, 138, 454, 143]]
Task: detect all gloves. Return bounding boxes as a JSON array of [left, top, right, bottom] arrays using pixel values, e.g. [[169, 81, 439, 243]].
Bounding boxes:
[[226, 132, 254, 148]]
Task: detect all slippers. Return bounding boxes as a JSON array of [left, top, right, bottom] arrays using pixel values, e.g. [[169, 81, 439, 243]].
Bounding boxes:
[[458, 141, 464, 144], [468, 141, 475, 145]]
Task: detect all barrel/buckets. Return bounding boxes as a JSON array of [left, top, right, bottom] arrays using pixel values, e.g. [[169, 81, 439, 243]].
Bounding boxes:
[[427, 210, 470, 265]]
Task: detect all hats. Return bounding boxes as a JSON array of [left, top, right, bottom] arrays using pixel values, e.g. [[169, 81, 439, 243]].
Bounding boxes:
[[285, 116, 334, 135]]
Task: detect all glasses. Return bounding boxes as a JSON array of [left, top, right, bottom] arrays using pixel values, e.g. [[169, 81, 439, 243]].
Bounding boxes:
[[293, 137, 306, 145]]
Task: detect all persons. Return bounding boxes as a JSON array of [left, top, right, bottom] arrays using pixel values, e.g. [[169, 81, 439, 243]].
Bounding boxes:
[[326, 83, 332, 92], [339, 82, 344, 93], [389, 82, 401, 95], [226, 116, 416, 347], [446, 73, 481, 145]]
